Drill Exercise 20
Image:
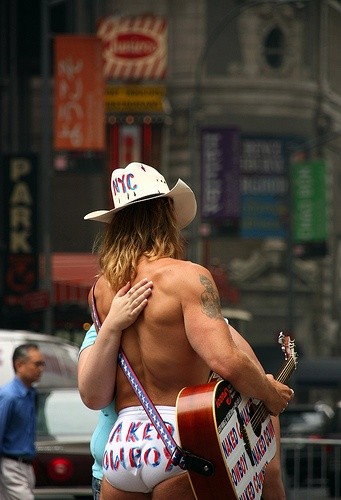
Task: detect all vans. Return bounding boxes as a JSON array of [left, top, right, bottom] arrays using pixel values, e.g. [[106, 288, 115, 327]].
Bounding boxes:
[[0, 331, 103, 499]]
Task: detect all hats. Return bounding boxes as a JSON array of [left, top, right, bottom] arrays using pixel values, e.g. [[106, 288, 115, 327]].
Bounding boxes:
[[83, 161, 197, 230]]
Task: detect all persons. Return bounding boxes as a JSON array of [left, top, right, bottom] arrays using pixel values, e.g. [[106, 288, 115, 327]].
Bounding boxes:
[[0, 344, 45, 500], [77, 161, 296, 500]]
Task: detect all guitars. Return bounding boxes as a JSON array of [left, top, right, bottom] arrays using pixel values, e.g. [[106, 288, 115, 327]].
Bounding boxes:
[[175, 332, 298, 500]]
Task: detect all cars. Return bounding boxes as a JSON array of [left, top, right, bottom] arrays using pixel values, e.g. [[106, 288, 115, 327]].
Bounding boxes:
[[279, 404, 341, 474]]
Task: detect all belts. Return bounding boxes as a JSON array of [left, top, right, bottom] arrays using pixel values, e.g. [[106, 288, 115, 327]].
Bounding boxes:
[[0, 451, 33, 465]]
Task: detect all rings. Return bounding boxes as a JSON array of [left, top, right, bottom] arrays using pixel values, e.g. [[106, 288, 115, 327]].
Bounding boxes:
[[285, 402, 288, 407], [129, 290, 132, 294], [280, 407, 285, 413], [290, 389, 294, 397]]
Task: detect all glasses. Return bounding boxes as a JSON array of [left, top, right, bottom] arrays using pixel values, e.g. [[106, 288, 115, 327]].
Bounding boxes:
[[20, 359, 46, 367]]
